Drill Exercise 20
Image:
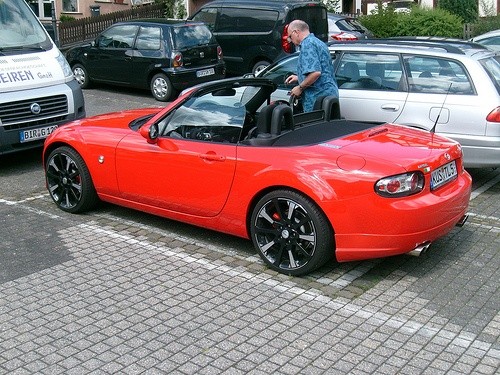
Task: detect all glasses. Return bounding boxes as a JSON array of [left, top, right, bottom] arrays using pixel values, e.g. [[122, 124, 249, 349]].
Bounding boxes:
[[289, 30, 300, 38]]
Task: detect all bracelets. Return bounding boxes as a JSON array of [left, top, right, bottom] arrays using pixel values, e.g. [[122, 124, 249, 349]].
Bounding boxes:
[[300, 86, 303, 88]]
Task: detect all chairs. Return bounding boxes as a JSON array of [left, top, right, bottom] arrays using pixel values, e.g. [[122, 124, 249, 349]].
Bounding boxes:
[[312, 97, 341, 122], [337, 61, 363, 90], [239, 101, 293, 146], [361, 61, 388, 89]]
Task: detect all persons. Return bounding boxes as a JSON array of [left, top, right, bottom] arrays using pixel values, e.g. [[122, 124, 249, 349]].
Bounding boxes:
[[285, 20, 338, 112]]
